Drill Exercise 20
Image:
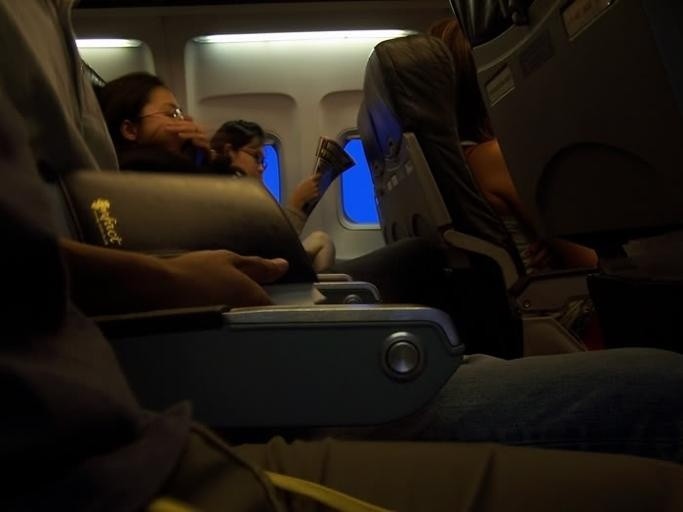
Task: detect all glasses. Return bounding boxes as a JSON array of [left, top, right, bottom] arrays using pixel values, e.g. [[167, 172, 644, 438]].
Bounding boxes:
[[239, 148, 263, 163], [140, 108, 184, 120]]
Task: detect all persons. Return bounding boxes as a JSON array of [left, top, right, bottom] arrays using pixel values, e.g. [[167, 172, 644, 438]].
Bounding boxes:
[[0, 0, 683, 466], [424, 14, 617, 332], [1, 91, 683, 511], [92, 70, 507, 362], [208, 120, 338, 276]]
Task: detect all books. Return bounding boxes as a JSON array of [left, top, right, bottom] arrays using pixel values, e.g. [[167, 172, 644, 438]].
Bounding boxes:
[[301, 136, 355, 220]]
